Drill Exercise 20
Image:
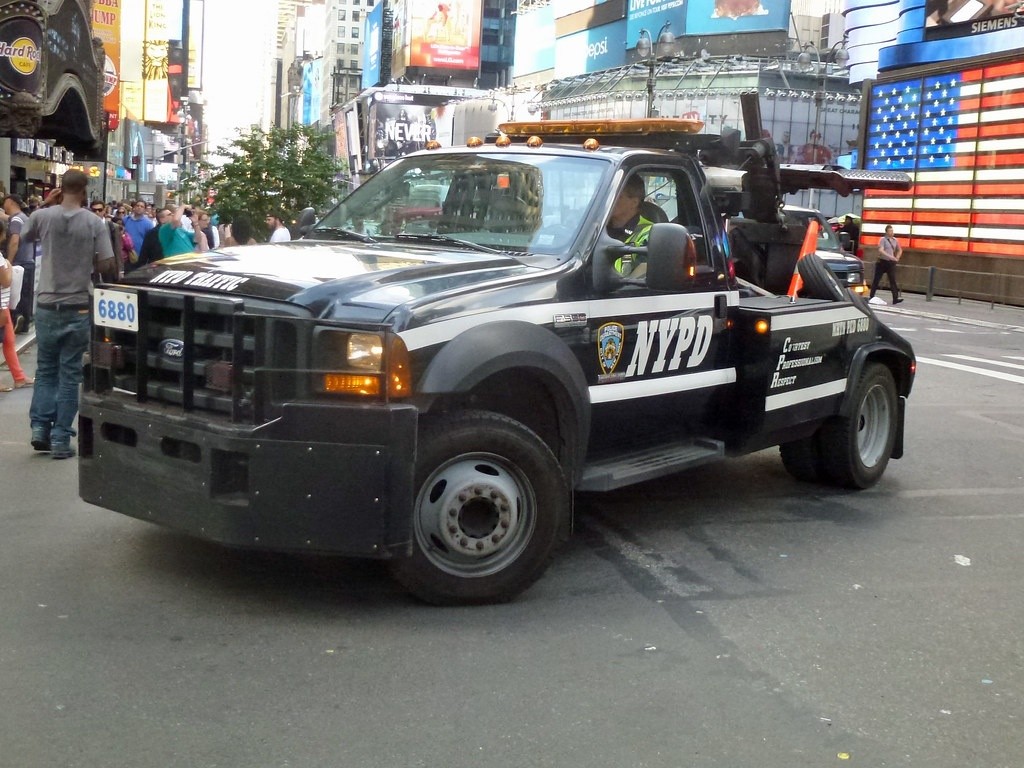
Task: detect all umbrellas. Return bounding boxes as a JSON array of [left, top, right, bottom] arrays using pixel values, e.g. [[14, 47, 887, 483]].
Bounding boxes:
[[827, 212, 862, 224]]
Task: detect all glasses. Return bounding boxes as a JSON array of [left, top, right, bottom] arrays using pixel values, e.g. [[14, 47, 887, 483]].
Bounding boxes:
[[118, 212, 125, 214], [92, 208, 102, 212]]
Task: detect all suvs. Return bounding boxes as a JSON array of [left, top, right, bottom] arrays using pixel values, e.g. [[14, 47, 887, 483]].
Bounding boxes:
[[77, 119, 918, 608], [777, 200, 870, 303]]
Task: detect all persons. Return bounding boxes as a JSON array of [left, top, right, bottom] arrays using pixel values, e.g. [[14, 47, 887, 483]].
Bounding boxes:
[[606, 174, 653, 277], [869, 226, 904, 305], [839, 216, 858, 249], [21, 169, 120, 459], [0, 193, 291, 391], [775, 131, 831, 165]]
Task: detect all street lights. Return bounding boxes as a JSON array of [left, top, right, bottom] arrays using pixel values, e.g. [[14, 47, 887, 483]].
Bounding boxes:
[[638, 21, 674, 121], [798, 38, 850, 210]]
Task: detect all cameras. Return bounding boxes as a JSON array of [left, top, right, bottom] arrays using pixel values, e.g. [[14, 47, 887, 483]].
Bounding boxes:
[[183, 209, 192, 217]]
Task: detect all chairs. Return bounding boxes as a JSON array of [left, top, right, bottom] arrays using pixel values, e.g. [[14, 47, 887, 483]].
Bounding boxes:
[[641, 202, 668, 224]]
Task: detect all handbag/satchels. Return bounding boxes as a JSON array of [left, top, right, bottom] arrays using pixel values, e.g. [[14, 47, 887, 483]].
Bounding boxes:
[[128, 249, 138, 263]]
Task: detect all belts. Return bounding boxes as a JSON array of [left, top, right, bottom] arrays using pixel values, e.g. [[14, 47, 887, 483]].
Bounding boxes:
[[37, 302, 87, 310]]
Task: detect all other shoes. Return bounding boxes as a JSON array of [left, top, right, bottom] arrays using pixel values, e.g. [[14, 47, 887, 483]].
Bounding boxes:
[[30, 426, 51, 451], [14, 376, 35, 388], [893, 299, 904, 305], [0, 385, 12, 392], [13, 315, 24, 334], [51, 440, 76, 458]]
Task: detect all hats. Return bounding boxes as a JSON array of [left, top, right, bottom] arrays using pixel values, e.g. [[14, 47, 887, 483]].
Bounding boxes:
[[3, 194, 23, 206], [267, 210, 284, 221]]
